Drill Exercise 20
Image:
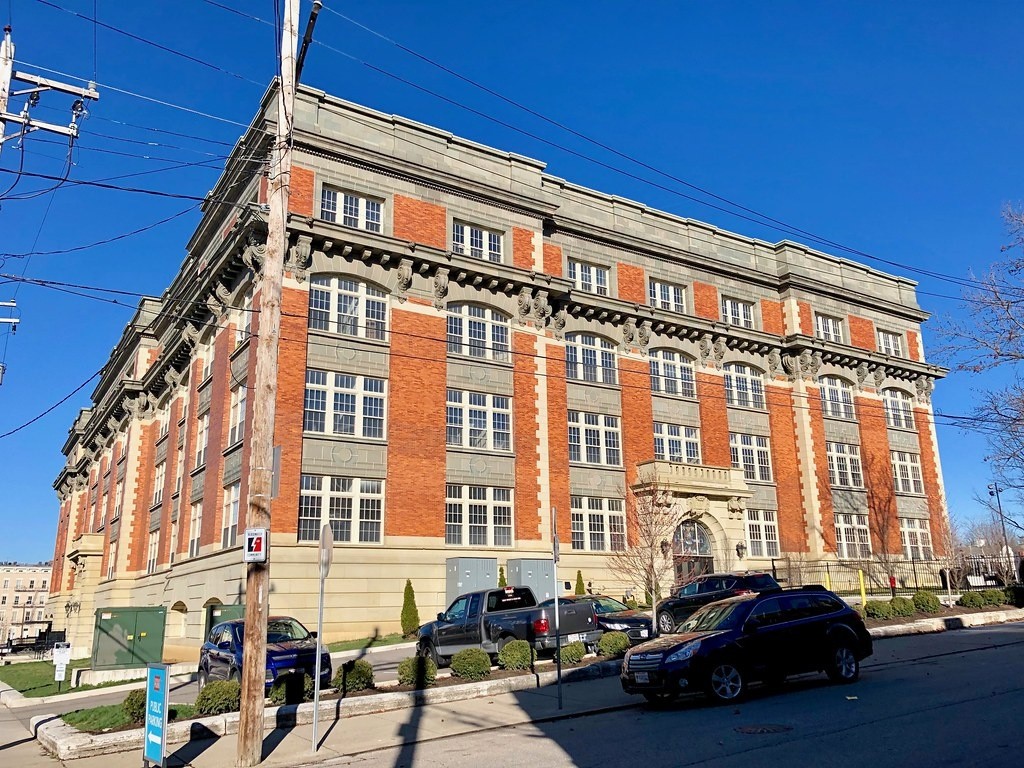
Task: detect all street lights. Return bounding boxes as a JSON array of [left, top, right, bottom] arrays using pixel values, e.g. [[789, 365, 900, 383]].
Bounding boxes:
[[987, 482, 1016, 578]]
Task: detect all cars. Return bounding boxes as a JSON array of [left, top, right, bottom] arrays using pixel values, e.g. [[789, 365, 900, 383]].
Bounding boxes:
[[537, 594, 660, 647], [197, 616, 332, 695]]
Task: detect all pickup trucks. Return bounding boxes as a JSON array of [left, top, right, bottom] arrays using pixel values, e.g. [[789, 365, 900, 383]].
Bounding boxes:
[[414, 585, 604, 668]]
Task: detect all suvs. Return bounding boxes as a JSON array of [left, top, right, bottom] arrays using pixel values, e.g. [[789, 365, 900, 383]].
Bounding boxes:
[[655, 571, 784, 634], [620, 584, 874, 706]]
